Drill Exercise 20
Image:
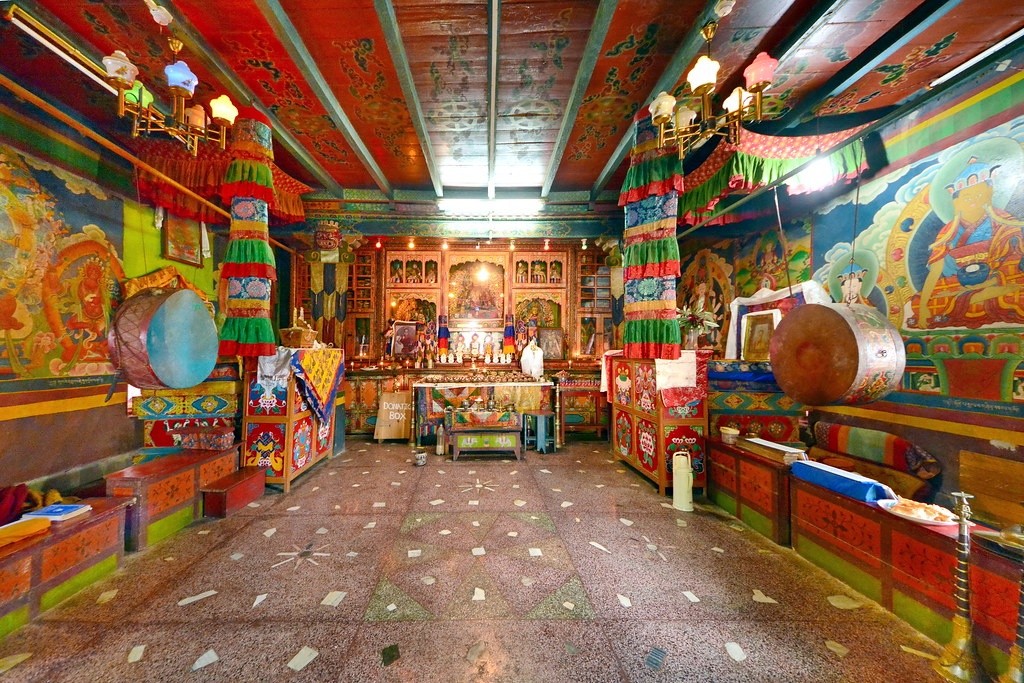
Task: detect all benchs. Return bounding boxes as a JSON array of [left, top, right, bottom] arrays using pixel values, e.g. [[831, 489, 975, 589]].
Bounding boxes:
[[202, 466, 273, 519]]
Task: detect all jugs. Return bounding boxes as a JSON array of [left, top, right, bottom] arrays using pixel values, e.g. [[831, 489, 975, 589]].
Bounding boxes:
[[672, 451, 693, 512]]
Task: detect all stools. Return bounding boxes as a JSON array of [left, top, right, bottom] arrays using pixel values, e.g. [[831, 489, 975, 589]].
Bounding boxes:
[[523, 410, 557, 453]]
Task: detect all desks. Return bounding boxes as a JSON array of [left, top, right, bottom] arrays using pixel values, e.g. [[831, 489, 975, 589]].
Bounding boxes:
[[374, 375, 610, 461], [0, 451, 238, 641], [704, 434, 1024, 655]]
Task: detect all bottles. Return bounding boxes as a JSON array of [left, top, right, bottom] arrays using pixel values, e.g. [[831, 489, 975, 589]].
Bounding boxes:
[[415, 446, 428, 467]]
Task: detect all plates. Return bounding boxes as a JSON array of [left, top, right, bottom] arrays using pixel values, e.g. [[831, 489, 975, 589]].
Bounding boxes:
[[876, 498, 960, 525]]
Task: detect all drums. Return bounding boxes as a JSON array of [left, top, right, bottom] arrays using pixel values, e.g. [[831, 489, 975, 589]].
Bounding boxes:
[[108, 286, 219, 389], [768, 303, 907, 408]]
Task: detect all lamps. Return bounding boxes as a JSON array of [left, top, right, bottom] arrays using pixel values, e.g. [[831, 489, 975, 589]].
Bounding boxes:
[[102, 29, 239, 157], [648, 23, 779, 160]]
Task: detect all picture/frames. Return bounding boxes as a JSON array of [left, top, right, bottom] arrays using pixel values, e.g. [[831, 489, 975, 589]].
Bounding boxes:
[[391, 321, 417, 356], [161, 208, 204, 268], [538, 327, 565, 360], [743, 313, 774, 363]]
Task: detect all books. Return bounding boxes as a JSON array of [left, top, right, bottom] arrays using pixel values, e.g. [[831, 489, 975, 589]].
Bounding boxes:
[[22, 505, 91, 521]]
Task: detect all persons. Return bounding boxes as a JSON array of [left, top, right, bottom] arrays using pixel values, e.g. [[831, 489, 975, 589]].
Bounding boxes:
[[396, 328, 415, 353]]
